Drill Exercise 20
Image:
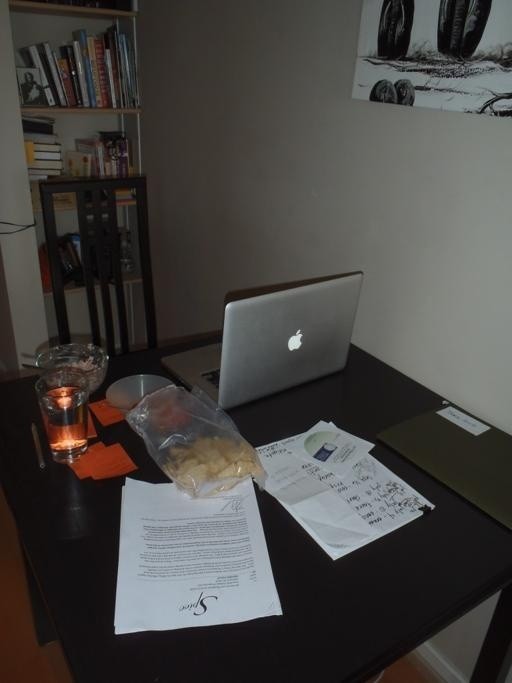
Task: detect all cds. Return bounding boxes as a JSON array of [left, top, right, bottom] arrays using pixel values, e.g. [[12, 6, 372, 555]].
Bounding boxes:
[[304, 430, 352, 464]]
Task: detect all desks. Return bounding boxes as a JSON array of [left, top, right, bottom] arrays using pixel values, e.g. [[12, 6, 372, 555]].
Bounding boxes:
[[0, 324, 512, 681]]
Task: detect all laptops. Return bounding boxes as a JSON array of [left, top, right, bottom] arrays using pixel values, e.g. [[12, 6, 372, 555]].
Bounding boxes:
[[161, 271, 364, 409]]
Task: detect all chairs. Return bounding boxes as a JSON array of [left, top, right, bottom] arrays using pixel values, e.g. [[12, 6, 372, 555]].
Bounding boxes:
[[37, 174, 161, 360]]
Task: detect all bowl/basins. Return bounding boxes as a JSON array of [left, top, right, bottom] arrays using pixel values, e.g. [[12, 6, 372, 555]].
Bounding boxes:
[[33, 340, 107, 396], [105, 373, 176, 411]]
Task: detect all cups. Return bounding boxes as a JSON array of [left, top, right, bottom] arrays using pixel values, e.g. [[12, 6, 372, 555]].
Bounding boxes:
[[33, 367, 89, 464]]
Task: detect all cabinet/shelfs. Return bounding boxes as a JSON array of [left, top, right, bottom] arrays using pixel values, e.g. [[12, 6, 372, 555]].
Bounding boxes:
[[9, 0, 152, 376]]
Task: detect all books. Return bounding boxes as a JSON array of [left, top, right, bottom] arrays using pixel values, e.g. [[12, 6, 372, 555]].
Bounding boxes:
[[16, 22, 135, 181]]
